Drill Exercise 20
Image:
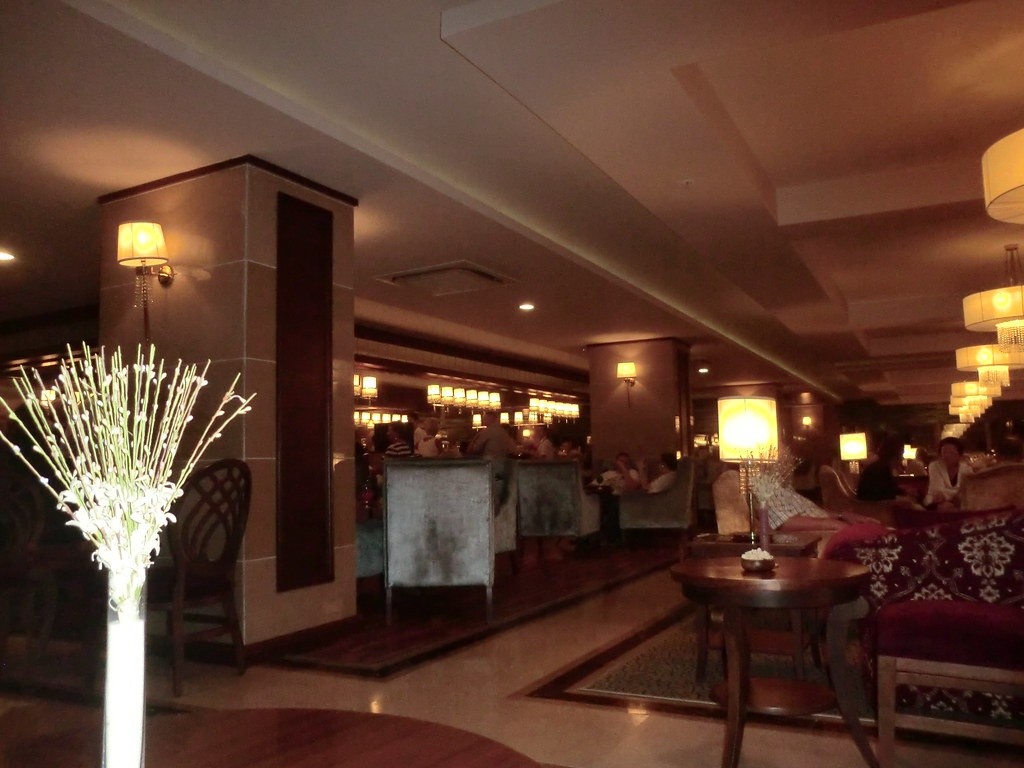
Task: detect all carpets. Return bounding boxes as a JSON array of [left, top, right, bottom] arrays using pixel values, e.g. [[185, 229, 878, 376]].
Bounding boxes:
[[508, 605, 878, 738]]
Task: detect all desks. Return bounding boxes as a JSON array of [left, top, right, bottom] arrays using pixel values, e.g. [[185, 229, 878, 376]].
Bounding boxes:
[[669, 555, 882, 768], [692, 531, 822, 683], [0, 707, 538, 768]]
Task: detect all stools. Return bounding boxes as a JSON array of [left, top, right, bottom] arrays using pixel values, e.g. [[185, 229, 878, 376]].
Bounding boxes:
[[878, 601, 1024, 768]]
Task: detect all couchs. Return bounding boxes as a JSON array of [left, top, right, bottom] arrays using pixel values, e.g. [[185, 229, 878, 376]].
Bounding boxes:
[[820, 506, 1024, 761]]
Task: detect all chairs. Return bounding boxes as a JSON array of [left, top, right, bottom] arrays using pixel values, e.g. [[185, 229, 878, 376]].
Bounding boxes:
[[382, 457, 497, 626], [352, 520, 385, 577], [0, 467, 59, 677], [619, 456, 700, 545], [495, 456, 521, 574], [78, 456, 252, 702], [517, 464, 601, 556]]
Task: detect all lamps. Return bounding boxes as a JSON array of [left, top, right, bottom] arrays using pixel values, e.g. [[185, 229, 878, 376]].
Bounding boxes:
[[962, 243, 1024, 354], [981, 128, 1024, 225], [353, 373, 580, 438], [948, 382, 1002, 423], [116, 218, 173, 313], [616, 361, 637, 384], [802, 417, 812, 428], [717, 395, 780, 568], [955, 343, 1024, 388], [839, 432, 868, 491]]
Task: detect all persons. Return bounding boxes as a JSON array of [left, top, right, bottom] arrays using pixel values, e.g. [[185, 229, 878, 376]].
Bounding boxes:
[[923, 437, 975, 509], [748, 444, 883, 554], [855, 438, 916, 504], [588, 451, 688, 543], [355, 410, 581, 510]]
